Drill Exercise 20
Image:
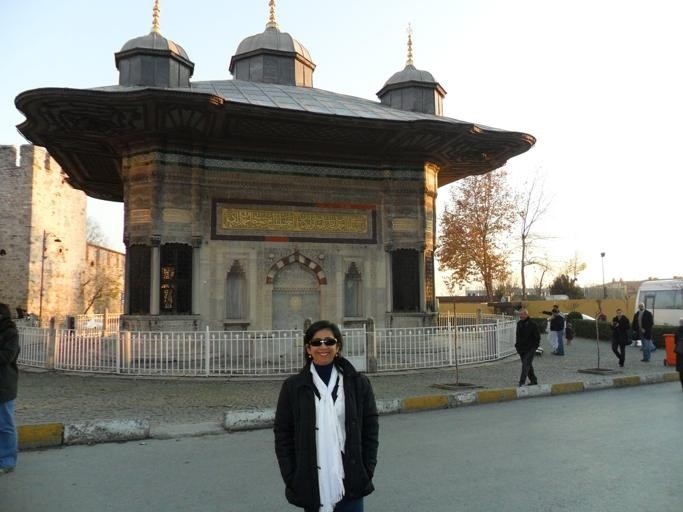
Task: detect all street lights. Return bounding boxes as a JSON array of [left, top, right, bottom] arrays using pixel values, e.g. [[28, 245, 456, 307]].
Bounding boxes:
[[38, 229, 62, 327], [600, 251, 608, 298]]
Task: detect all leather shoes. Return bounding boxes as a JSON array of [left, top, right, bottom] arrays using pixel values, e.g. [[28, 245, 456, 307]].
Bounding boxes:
[[520, 382, 537, 387]]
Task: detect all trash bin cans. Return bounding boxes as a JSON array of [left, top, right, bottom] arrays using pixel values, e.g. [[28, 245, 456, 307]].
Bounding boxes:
[[662, 334, 676, 367]]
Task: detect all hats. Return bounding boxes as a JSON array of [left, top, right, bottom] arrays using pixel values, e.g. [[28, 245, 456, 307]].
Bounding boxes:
[[553, 309, 559, 313]]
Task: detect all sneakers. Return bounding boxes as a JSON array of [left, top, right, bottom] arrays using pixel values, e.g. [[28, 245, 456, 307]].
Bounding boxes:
[[0, 467, 14, 475]]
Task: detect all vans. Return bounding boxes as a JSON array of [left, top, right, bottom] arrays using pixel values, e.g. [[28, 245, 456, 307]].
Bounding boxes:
[[635, 279, 683, 349]]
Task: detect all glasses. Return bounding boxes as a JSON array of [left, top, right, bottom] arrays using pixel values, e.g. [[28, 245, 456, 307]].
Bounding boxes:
[[310, 338, 337, 346]]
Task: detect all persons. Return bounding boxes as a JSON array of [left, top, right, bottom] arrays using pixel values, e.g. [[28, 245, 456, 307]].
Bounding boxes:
[[610, 308, 630, 367], [545, 305, 566, 349], [273, 320, 379, 512], [632, 303, 654, 362], [515, 309, 541, 387], [16, 305, 27, 318], [0, 303, 21, 475], [674, 318, 683, 389], [550, 309, 564, 356]]
